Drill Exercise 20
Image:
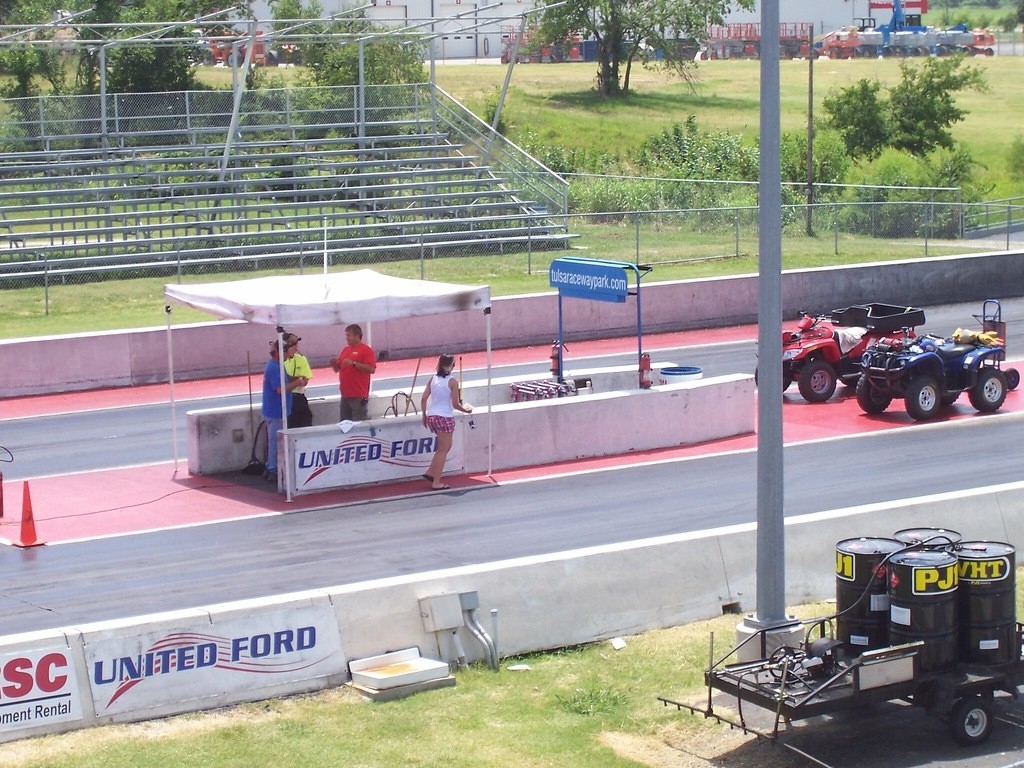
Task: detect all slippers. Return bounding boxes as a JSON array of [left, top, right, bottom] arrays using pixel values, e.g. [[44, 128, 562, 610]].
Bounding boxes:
[[422, 474, 433, 482], [433, 484, 450, 489]]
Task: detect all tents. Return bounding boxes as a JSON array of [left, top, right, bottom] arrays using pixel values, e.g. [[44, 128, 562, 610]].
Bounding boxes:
[[163, 269, 492, 499]]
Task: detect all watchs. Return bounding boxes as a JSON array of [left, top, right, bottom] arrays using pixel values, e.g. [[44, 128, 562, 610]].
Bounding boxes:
[[353, 361, 356, 367]]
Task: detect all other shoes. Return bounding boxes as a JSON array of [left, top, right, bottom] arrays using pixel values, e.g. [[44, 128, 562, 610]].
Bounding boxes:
[[268, 473, 276, 480], [263, 470, 269, 478]]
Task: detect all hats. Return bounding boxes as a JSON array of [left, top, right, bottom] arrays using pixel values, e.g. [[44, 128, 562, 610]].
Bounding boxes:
[[286, 334, 301, 346], [269, 339, 291, 352]]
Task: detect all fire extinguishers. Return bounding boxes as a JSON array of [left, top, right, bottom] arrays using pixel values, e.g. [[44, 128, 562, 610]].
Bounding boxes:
[[548, 338, 568, 374], [638, 352, 653, 386]]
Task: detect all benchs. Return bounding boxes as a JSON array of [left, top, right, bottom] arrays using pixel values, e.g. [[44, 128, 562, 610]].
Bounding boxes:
[[0, 118, 582, 277]]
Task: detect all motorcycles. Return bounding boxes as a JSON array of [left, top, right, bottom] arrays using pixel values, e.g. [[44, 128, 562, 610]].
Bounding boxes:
[[754, 302, 926, 404], [854, 299, 1020, 422]]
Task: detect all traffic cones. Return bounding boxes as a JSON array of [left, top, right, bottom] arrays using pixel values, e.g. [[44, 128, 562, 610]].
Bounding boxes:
[[13, 479, 45, 547]]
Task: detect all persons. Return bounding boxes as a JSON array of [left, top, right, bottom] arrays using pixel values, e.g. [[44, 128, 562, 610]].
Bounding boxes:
[[262, 332, 313, 481], [421, 354, 473, 490], [329, 324, 377, 422]]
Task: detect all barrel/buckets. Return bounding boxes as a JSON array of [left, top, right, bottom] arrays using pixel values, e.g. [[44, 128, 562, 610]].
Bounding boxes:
[[834, 526, 1017, 672], [659, 366, 704, 385]]
